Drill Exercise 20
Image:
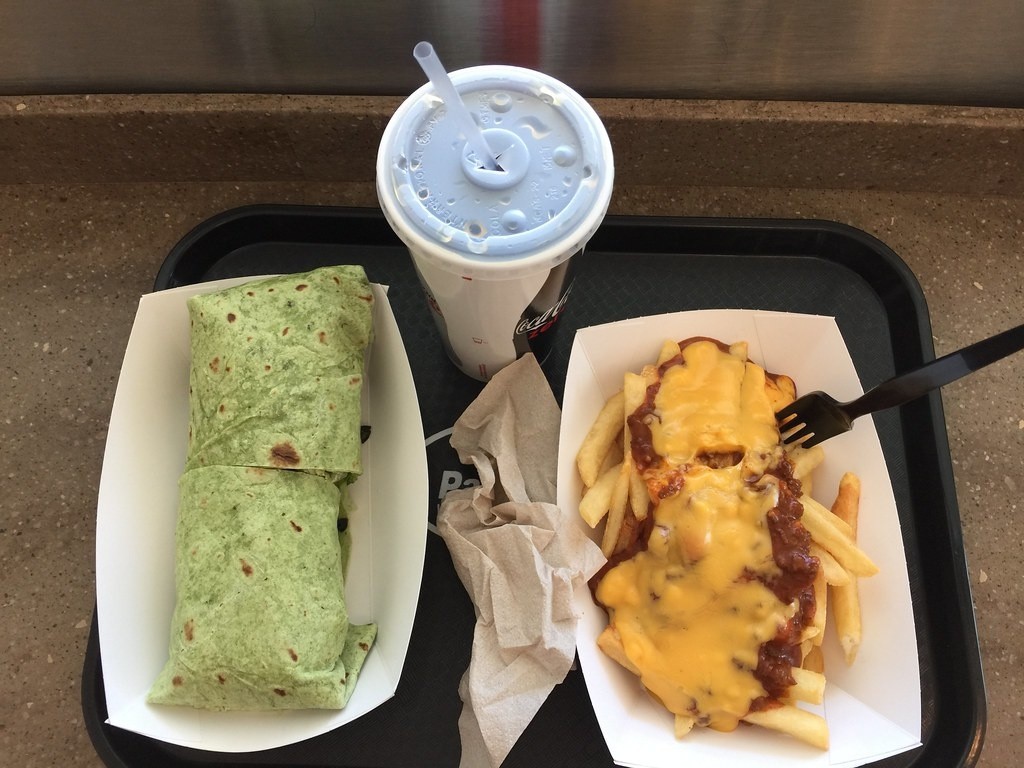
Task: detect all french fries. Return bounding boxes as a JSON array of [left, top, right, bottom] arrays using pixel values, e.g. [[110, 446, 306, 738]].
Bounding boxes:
[[577, 340, 880, 753]]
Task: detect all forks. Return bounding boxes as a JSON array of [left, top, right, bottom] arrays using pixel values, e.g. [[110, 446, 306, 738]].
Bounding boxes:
[[782, 325, 1024, 445]]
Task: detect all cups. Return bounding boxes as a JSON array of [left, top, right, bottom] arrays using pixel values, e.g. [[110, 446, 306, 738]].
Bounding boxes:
[[374, 67, 615, 389]]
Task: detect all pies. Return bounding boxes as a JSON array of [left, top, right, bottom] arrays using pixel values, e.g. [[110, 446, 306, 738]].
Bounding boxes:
[[147, 264, 377, 708]]
